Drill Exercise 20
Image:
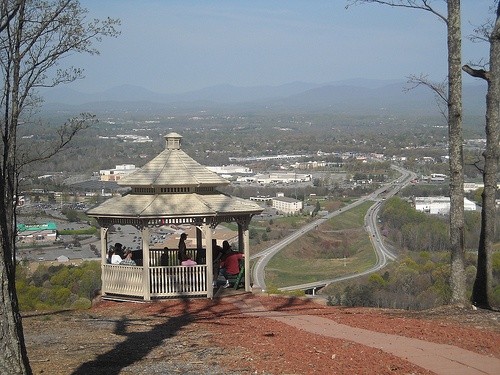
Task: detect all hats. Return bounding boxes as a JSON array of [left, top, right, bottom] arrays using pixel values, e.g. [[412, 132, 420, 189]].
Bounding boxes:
[[181, 233, 188, 237]]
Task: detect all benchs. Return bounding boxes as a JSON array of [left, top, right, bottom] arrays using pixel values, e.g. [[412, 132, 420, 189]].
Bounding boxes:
[[226, 259, 245, 291]]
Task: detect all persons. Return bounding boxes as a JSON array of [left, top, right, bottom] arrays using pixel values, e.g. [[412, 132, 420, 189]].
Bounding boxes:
[[220, 246, 245, 289], [177, 233, 188, 264], [182, 253, 196, 265], [120, 250, 136, 265], [108, 243, 126, 264], [212, 239, 221, 259], [213, 241, 229, 288], [161, 247, 169, 265]]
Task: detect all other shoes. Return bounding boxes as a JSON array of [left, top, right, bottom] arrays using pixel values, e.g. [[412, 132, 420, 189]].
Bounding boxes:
[[212, 284, 217, 288], [222, 283, 230, 288]]
